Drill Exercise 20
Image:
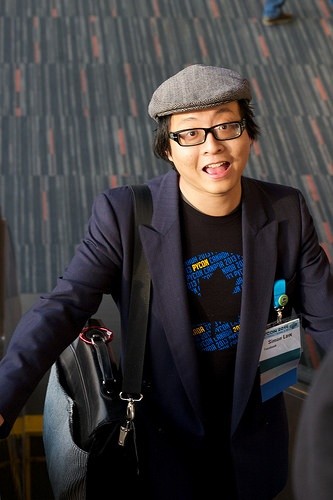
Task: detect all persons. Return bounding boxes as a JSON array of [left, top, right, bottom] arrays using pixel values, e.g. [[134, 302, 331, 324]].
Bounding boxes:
[[0, 63, 333, 500], [263, 0, 291, 26]]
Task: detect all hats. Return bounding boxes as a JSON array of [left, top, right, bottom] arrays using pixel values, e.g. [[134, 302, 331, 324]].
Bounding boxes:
[[148, 63, 253, 125]]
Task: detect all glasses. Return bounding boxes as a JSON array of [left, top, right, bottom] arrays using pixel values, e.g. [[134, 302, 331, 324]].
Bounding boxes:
[[168, 118, 246, 148]]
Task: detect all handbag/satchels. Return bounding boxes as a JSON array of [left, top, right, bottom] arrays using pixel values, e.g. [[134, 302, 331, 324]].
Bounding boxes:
[[42, 184, 151, 500]]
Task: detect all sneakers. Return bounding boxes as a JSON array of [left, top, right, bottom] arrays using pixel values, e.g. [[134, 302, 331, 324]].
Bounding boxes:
[[262, 15, 295, 25]]
